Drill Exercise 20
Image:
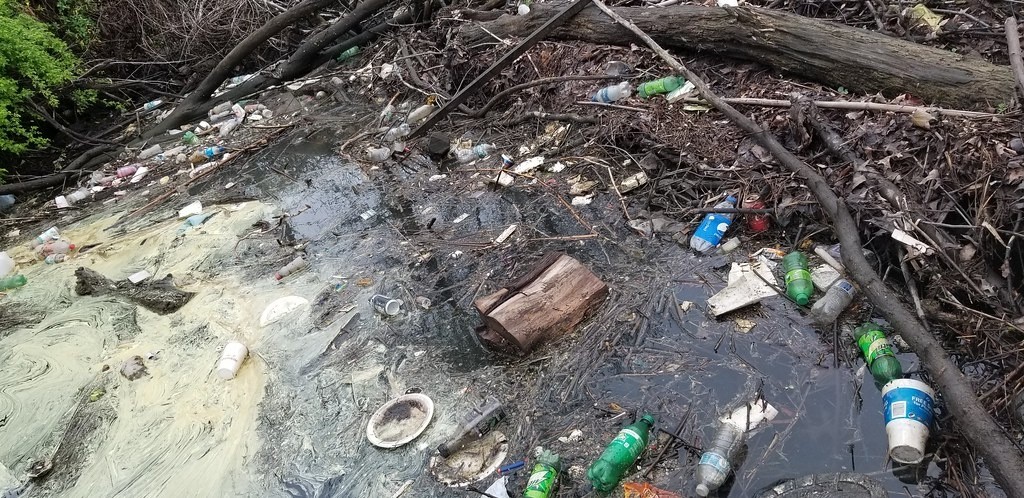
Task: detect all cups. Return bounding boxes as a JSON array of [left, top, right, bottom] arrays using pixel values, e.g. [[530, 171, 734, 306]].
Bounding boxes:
[[882, 378, 937, 462], [216, 343, 247, 380]]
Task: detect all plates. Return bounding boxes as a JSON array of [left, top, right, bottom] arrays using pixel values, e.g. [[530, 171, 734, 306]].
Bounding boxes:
[[260, 296, 312, 325], [366, 393, 434, 450], [431, 432, 510, 487]]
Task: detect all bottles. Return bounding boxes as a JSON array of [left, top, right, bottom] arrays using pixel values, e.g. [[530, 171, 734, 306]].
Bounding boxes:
[[524, 447, 562, 498], [585, 196, 902, 498], [636, 77, 684, 98], [591, 82, 634, 104], [436, 396, 505, 456], [0, 44, 498, 290]]
